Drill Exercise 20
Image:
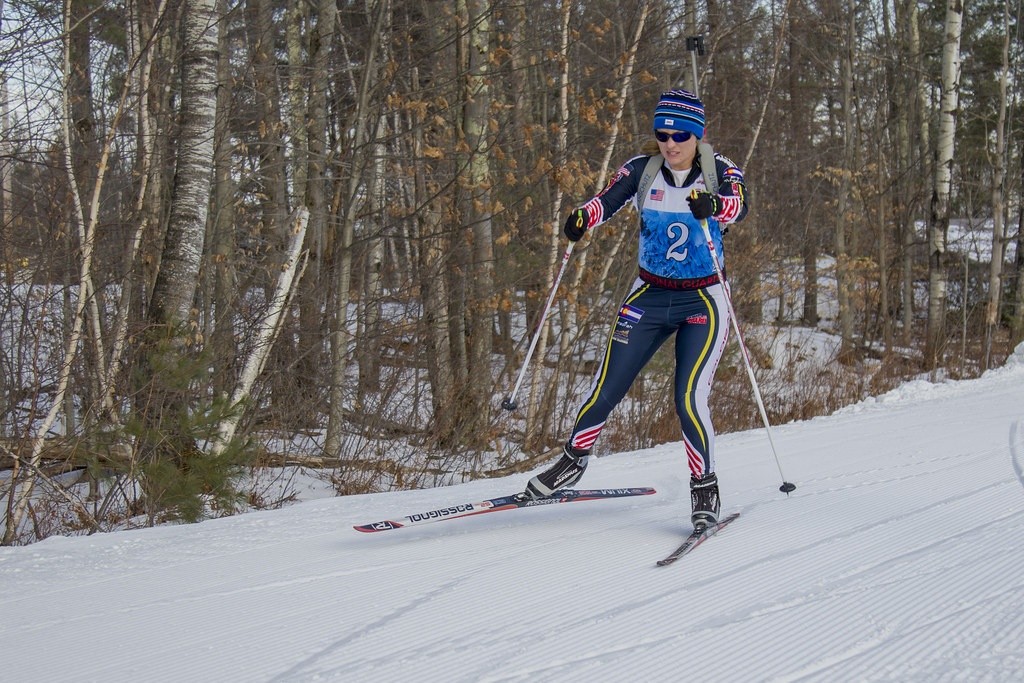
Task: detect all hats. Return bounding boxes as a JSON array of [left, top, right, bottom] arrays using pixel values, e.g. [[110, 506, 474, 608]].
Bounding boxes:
[[653, 89, 705, 140]]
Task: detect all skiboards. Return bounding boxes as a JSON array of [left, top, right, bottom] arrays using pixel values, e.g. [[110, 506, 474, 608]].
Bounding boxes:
[[352, 487, 741, 570]]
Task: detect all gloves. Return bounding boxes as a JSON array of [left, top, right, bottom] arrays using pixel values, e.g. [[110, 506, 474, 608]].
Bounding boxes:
[[686, 190, 723, 220], [564, 207, 590, 241]]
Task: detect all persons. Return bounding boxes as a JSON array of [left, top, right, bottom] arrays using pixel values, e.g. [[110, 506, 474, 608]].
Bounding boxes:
[[523, 88, 749, 530]]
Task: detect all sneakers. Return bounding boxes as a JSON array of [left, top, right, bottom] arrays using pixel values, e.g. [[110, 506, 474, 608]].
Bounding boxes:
[[689, 472, 721, 529], [525, 440, 590, 500]]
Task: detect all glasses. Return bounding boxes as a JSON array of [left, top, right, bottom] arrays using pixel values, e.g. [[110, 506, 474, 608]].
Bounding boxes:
[[654, 129, 692, 143]]
